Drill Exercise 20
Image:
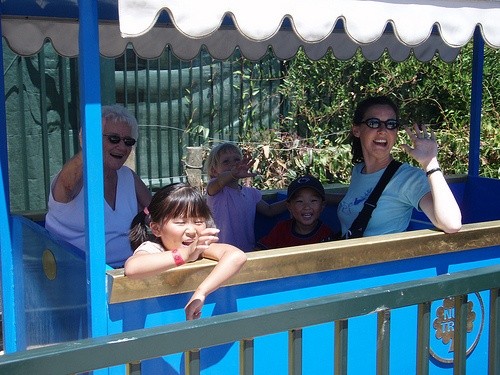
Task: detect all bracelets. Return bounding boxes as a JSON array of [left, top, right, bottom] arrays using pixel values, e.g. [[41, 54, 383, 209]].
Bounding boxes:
[[171, 249, 185, 266], [426, 168, 441, 175], [230, 173, 237, 181]]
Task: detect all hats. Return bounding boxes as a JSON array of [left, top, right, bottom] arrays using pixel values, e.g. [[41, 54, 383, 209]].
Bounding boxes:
[[287, 175, 326, 202]]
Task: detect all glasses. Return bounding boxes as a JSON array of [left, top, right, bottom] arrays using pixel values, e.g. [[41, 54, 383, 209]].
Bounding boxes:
[[102, 134, 138, 146], [360, 118, 400, 130]]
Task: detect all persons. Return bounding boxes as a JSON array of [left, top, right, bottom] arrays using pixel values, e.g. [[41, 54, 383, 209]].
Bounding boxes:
[[124, 183, 247, 320], [203, 143, 289, 252], [325, 96, 462, 240], [249, 175, 334, 252], [45, 105, 151, 268]]
